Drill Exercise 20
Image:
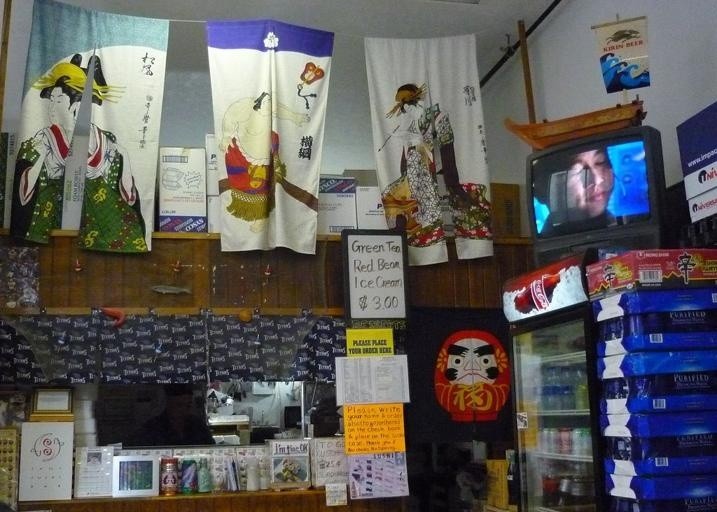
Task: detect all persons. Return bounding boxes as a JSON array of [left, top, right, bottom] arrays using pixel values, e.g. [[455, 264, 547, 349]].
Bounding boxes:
[[123, 384, 216, 446], [384, 83, 491, 248], [531, 146, 617, 239], [218, 93, 318, 232], [10, 53, 148, 254]]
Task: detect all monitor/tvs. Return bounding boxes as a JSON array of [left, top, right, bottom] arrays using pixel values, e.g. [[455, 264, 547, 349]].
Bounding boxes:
[[526, 125, 669, 267]]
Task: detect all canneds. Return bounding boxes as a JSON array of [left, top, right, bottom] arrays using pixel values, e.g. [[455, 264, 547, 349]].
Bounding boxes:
[[181, 459, 198, 494], [536, 427, 592, 454], [161, 458, 179, 495]]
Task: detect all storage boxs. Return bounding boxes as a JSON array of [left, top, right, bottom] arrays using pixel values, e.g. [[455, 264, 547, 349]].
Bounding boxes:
[[318, 174, 358, 234], [158, 148, 206, 233], [205, 131, 222, 196], [344, 170, 399, 230], [436, 175, 457, 229], [207, 196, 221, 234], [489, 182, 521, 233]]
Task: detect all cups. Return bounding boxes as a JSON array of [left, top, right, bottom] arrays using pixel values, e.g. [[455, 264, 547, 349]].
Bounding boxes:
[[160, 457, 197, 496]]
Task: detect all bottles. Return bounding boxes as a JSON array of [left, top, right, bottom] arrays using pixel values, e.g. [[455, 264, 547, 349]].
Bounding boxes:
[[532, 364, 591, 408], [507, 453, 519, 505], [542, 459, 596, 511], [197, 456, 211, 493], [515, 267, 571, 313]]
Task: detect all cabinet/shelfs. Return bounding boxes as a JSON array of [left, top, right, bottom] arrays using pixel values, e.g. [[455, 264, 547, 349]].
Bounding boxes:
[[206, 238, 339, 313], [0, 241, 191, 311], [402, 246, 530, 311]]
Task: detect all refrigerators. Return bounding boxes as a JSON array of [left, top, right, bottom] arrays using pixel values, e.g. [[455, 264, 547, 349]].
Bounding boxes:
[[498, 246, 606, 512]]
